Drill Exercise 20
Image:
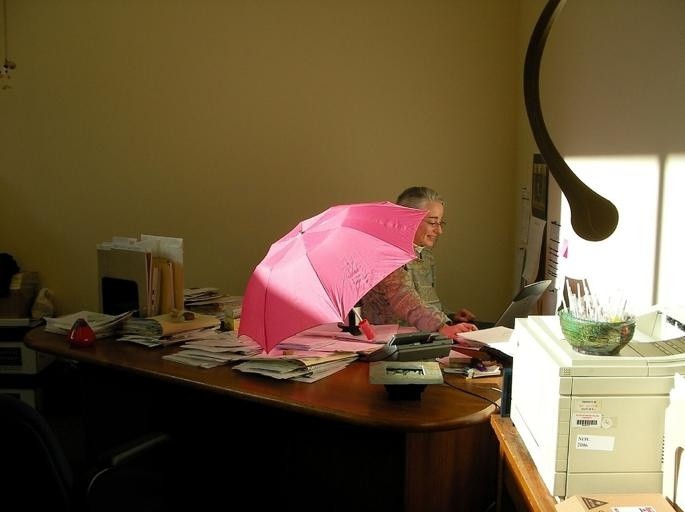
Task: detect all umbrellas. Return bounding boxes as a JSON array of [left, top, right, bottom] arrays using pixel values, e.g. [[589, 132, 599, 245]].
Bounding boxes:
[[238, 202, 432, 357]]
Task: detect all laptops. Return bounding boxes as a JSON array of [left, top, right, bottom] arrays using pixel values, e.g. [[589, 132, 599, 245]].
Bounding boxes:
[[448, 280, 553, 330]]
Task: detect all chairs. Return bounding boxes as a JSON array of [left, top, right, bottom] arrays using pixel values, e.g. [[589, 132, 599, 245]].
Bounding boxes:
[[0, 391, 170, 511]]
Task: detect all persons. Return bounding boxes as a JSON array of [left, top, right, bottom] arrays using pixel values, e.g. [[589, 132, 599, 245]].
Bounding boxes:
[[362, 186, 480, 343]]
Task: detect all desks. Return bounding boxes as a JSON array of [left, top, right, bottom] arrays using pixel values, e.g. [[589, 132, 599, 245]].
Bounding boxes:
[[22, 319, 685, 511]]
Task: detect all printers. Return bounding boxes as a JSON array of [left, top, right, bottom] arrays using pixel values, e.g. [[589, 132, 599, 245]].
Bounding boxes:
[[510, 304, 685, 497]]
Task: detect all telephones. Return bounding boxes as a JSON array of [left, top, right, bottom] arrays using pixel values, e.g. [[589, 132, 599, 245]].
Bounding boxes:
[[367, 331, 454, 362]]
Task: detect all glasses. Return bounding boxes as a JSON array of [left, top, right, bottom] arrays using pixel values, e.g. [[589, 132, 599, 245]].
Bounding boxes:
[[423, 220, 446, 231]]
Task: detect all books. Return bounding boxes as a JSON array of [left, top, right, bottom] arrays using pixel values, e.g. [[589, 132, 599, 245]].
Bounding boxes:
[[41, 233, 400, 385], [457, 325, 515, 360]]
